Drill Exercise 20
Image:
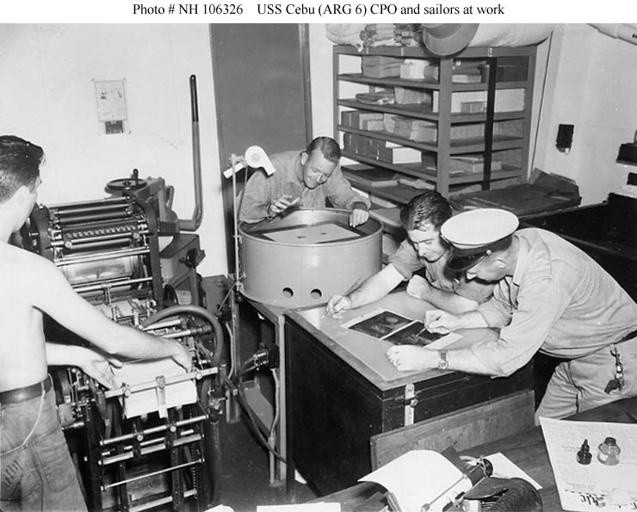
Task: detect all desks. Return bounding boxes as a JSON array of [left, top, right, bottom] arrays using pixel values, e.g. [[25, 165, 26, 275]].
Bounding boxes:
[[302, 395, 636, 512]]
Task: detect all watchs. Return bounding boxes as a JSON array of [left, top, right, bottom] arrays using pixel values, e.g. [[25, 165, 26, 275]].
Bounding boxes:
[[437, 348, 450, 372]]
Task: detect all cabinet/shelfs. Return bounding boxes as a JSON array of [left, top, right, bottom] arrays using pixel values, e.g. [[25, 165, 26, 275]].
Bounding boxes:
[[331, 43, 536, 266]]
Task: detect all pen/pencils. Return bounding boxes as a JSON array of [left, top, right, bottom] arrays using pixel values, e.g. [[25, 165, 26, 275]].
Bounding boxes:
[[325, 277, 361, 318], [415, 315, 442, 338]]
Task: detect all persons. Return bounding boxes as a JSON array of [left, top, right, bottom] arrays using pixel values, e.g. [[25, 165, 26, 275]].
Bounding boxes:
[[0, 134, 195, 512], [325, 188, 494, 321], [233, 136, 371, 231], [385, 206, 636, 426]]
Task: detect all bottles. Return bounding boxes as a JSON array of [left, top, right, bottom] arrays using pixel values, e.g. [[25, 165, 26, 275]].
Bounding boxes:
[[576, 436, 621, 465]]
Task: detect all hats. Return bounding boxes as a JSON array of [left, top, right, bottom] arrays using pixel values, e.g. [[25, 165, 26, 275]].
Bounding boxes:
[[440, 207, 520, 273]]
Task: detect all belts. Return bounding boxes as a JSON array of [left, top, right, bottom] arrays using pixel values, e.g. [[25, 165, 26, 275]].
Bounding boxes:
[[0, 374, 55, 405], [613, 330, 635, 345]]
